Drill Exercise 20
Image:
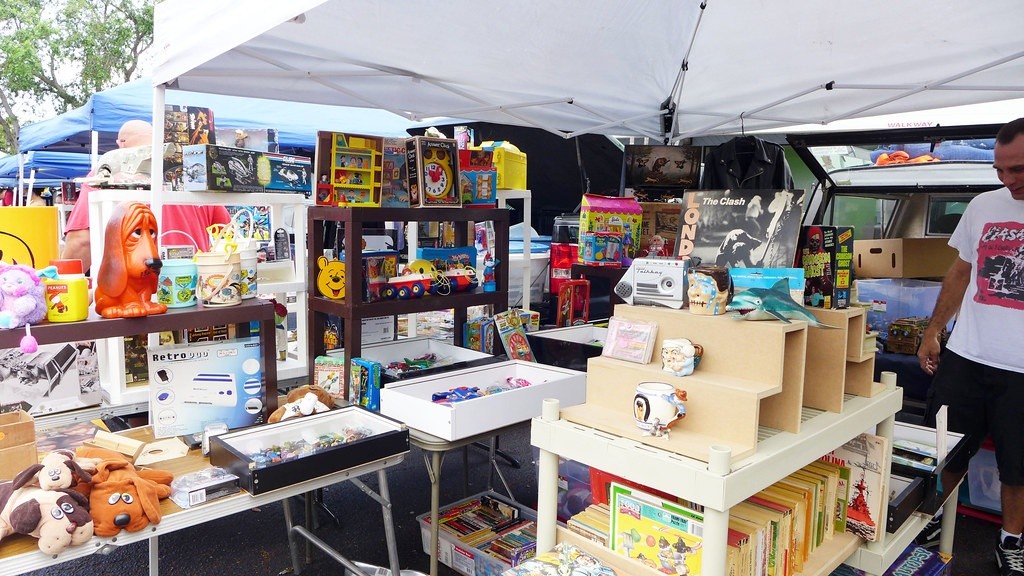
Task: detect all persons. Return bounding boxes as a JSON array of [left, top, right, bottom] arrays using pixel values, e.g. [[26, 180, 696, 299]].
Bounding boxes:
[[917, 118, 1024, 576], [26, 187, 46, 206], [0, 187, 26, 206], [641, 235, 668, 257], [63, 119, 232, 429]]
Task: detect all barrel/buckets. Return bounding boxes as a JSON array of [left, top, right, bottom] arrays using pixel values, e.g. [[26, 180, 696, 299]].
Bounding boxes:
[[155, 230, 198, 309], [195, 222, 242, 308], [215, 209, 257, 299]]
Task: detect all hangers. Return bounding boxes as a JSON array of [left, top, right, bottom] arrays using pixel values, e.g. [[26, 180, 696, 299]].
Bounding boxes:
[[735, 111, 754, 154]]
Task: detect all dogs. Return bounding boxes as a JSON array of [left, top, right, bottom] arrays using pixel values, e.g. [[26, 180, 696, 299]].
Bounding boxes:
[[93, 204, 168, 318]]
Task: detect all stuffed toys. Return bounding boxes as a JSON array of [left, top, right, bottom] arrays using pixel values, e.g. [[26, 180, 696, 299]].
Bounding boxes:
[[268, 384, 333, 424], [0, 449, 174, 555], [0, 260, 47, 353]]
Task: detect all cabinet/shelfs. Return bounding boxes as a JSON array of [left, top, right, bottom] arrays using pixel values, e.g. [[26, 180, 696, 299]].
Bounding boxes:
[[531, 307, 968, 576], [88, 189, 308, 404], [308, 206, 510, 401]]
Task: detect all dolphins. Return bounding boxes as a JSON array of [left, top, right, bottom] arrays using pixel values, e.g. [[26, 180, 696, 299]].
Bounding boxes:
[[726, 277, 844, 330]]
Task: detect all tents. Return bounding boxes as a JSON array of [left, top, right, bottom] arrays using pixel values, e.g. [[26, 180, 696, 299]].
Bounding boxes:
[[0, 76, 419, 206], [149, 0, 1024, 576]]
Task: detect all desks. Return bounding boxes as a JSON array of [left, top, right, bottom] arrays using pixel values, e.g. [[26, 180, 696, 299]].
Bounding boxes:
[[571, 264, 630, 317], [305, 399, 530, 576], [0, 394, 405, 576]]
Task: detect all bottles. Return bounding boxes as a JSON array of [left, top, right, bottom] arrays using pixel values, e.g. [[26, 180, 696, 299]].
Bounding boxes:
[[46, 259, 88, 322]]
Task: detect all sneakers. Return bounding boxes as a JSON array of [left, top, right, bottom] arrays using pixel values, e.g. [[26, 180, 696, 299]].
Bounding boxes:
[[911, 514, 942, 547], [994, 528, 1024, 576]]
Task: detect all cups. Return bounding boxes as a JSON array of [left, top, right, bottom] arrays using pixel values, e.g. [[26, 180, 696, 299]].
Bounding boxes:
[[662, 339, 703, 377], [687, 266, 735, 316], [632, 382, 688, 438]]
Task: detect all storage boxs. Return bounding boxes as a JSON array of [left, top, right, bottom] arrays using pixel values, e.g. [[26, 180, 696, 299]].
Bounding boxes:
[[313, 130, 527, 208], [183, 143, 312, 194], [827, 421, 1002, 576], [795, 225, 959, 355], [0, 409, 37, 480], [508, 193, 643, 327], [146, 337, 262, 438], [208, 404, 409, 496], [327, 337, 591, 576]]
